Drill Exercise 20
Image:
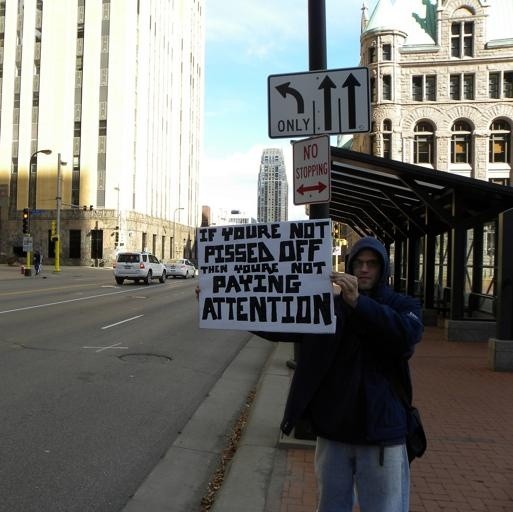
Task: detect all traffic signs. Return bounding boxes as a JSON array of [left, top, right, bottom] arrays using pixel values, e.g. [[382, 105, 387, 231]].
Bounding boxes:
[[264, 65, 370, 140]]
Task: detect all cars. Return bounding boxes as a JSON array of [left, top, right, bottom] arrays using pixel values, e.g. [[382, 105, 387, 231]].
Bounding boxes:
[[163, 258, 195, 279]]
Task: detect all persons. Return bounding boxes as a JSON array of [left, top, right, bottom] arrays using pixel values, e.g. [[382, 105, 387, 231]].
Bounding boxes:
[[33, 248, 44, 275], [194, 236, 428, 512]]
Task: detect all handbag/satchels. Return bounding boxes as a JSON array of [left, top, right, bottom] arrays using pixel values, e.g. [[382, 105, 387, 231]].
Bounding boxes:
[[406, 406, 426, 457]]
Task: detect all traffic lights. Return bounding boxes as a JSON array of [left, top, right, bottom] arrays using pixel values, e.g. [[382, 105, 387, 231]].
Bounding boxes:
[[50, 235, 58, 241], [291, 134, 330, 205], [114, 227, 118, 246], [89, 205, 93, 212], [21, 207, 29, 234]]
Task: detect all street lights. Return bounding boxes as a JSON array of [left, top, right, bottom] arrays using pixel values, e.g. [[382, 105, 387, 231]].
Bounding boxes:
[[24, 150, 52, 277], [173, 207, 184, 259]]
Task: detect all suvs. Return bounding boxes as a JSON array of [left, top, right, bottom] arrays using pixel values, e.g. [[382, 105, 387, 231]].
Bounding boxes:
[[112, 252, 167, 286]]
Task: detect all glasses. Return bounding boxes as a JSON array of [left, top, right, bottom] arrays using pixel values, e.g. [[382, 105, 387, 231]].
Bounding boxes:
[[350, 259, 379, 269]]
